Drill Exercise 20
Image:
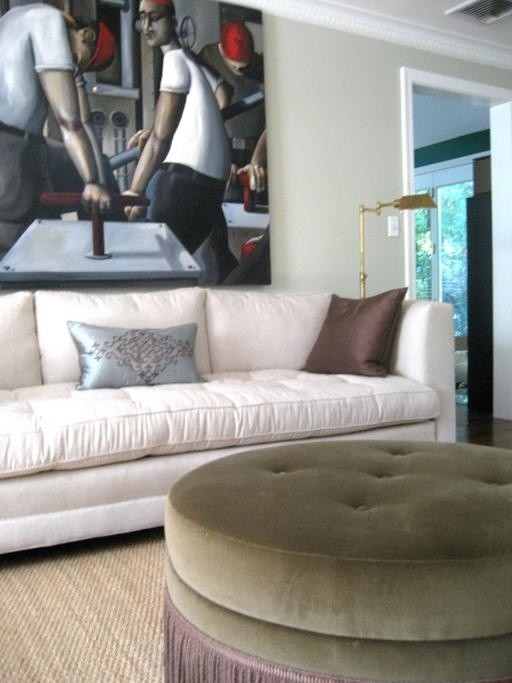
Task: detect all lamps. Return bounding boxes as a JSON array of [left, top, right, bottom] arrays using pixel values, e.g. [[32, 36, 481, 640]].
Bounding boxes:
[[358, 193, 437, 299]]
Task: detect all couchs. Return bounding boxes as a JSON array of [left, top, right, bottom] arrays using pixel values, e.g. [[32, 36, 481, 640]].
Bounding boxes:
[[162, 438, 509, 683], [0, 281, 457, 555]]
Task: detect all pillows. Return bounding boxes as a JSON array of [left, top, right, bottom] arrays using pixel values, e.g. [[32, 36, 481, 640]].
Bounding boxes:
[[0, 290, 43, 389], [65, 322, 207, 390], [301, 287, 408, 379], [35, 285, 210, 386], [206, 288, 339, 374]]
